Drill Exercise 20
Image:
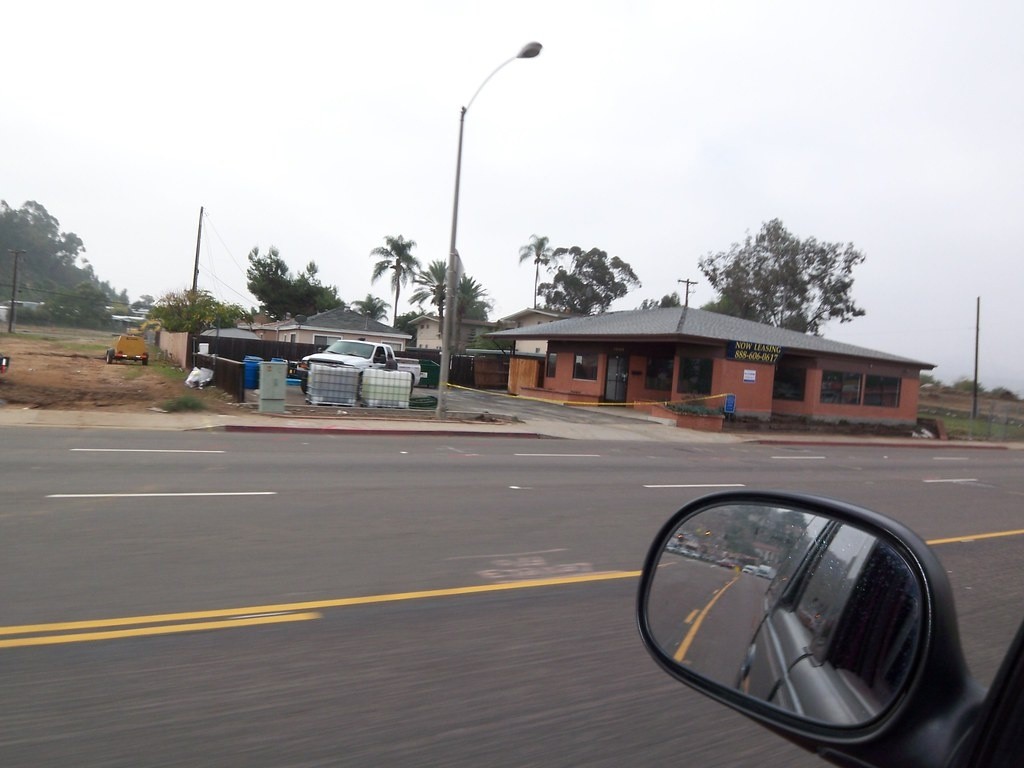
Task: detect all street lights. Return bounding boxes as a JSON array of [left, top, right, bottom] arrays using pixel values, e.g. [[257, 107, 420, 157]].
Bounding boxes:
[[433, 39, 544, 416], [9, 248, 27, 333]]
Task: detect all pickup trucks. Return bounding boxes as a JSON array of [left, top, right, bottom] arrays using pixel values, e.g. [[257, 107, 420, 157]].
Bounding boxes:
[[295, 337, 421, 397]]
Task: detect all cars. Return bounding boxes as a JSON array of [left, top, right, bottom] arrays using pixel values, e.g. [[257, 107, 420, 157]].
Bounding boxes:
[[666, 537, 777, 581]]
